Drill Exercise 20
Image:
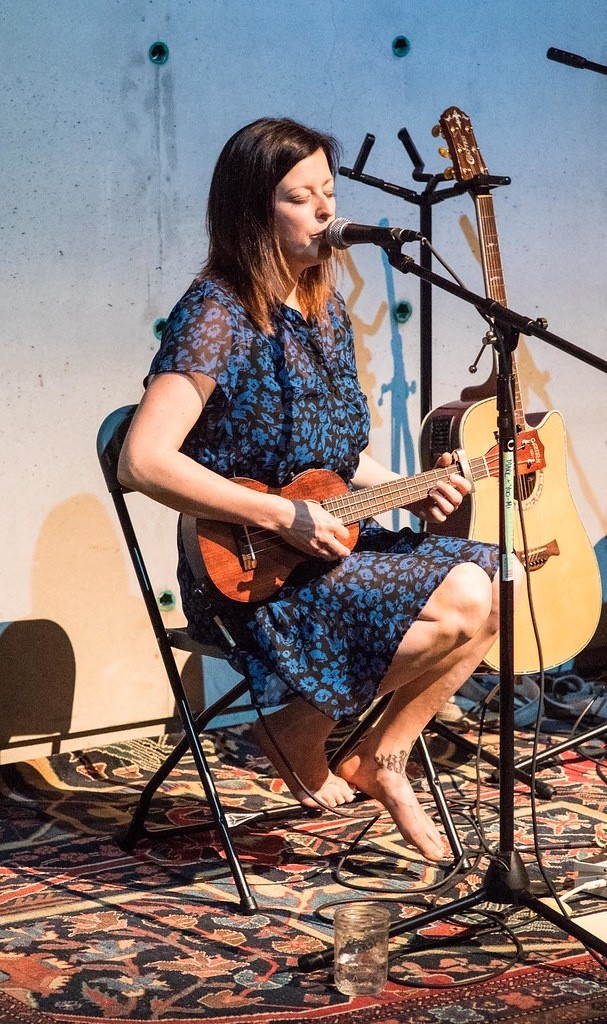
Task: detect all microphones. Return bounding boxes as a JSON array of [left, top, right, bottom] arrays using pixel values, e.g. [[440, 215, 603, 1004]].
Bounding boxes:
[[324, 217, 425, 252]]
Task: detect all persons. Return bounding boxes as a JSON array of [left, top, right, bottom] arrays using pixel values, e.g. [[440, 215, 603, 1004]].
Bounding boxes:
[[117, 118, 520, 863]]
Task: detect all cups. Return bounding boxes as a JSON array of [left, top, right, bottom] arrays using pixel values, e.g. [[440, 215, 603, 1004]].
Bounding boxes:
[[333, 905, 391, 996]]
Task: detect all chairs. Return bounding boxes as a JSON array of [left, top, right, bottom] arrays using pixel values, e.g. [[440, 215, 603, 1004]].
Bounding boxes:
[[95, 401, 469, 911]]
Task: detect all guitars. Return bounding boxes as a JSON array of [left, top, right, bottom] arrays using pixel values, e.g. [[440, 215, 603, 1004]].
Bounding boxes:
[[418, 107, 602, 675], [181, 424, 547, 603]]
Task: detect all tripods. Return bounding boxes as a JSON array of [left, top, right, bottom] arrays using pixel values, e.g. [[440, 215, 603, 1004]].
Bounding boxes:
[[298, 129, 606, 973]]
